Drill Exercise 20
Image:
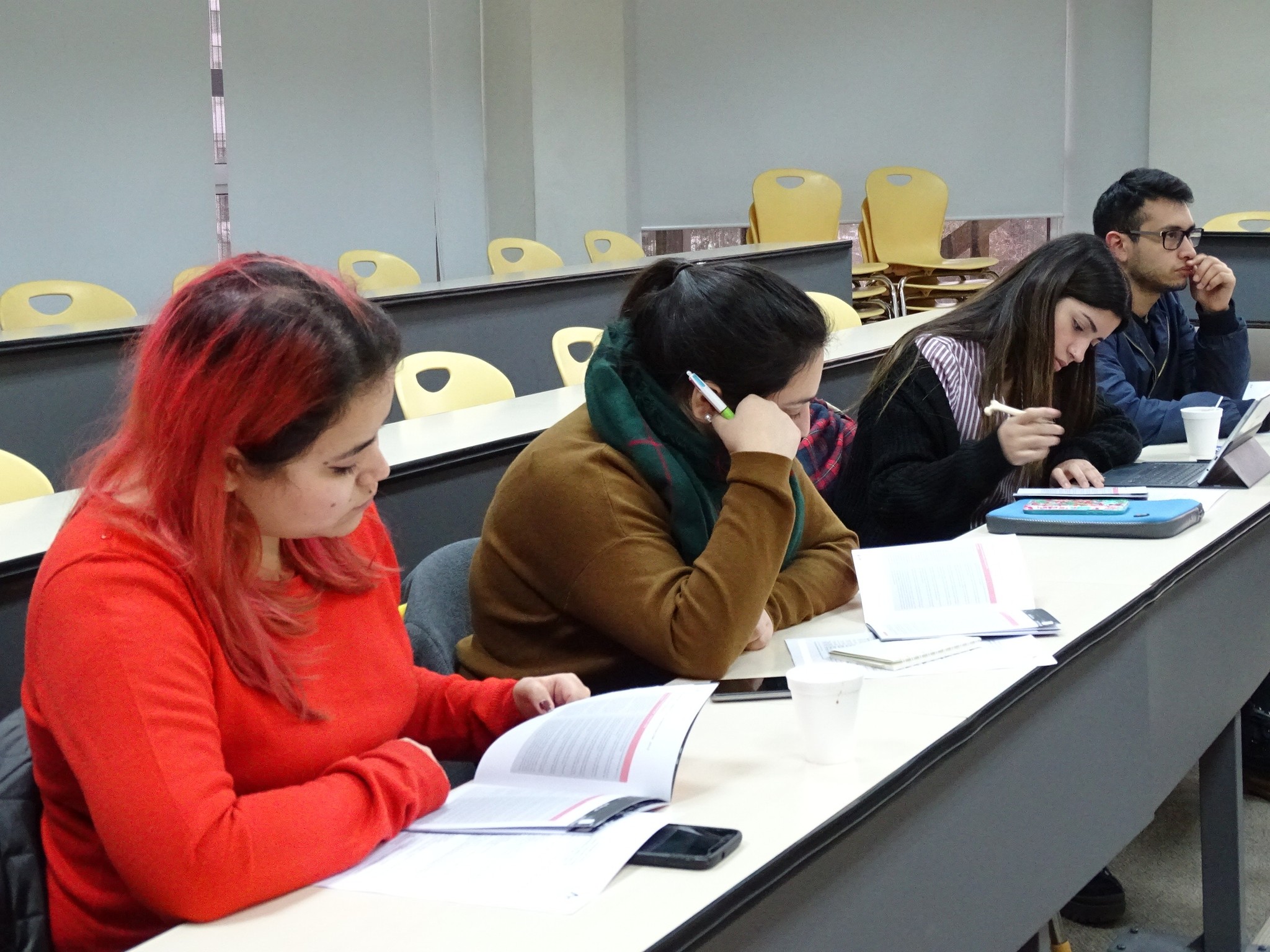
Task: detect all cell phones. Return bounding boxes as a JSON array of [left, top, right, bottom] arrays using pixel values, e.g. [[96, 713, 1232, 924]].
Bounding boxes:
[[627, 824, 742, 871], [711, 676, 792, 702], [1023, 499, 1128, 515]]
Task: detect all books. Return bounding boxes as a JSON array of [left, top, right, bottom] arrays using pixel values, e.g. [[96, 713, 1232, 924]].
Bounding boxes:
[[845, 532, 1039, 642], [828, 631, 986, 670], [964, 606, 1062, 637], [1011, 483, 1149, 500], [398, 674, 723, 839]]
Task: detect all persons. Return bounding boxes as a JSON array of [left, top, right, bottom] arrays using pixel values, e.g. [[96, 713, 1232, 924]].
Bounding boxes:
[[468, 256, 857, 690], [1075, 167, 1269, 440], [824, 231, 1145, 537], [22, 252, 592, 950]]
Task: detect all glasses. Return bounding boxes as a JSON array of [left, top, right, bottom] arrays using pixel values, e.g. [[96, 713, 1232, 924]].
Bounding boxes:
[[1127, 228, 1206, 250]]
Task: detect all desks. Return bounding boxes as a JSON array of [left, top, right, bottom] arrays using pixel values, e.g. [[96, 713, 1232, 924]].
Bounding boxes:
[[0, 309, 956, 723], [121, 380, 1270, 952], [0, 239, 853, 495]]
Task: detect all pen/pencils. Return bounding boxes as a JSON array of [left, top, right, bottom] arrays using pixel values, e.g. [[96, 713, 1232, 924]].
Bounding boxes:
[[984, 400, 1056, 424], [687, 370, 735, 420]]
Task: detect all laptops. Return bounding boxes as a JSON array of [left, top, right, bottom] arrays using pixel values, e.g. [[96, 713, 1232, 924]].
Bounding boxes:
[[1098, 394, 1270, 486]]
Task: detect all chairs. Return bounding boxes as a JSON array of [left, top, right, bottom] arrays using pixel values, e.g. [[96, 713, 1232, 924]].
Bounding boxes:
[[1204, 211, 1270, 232], [0, 163, 1001, 952]]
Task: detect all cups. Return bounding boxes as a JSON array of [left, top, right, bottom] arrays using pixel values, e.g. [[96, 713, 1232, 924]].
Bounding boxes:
[[1180, 407, 1224, 461]]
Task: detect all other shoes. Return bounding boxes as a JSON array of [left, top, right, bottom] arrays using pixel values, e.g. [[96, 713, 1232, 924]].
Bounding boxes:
[[1060, 865, 1125, 928]]
